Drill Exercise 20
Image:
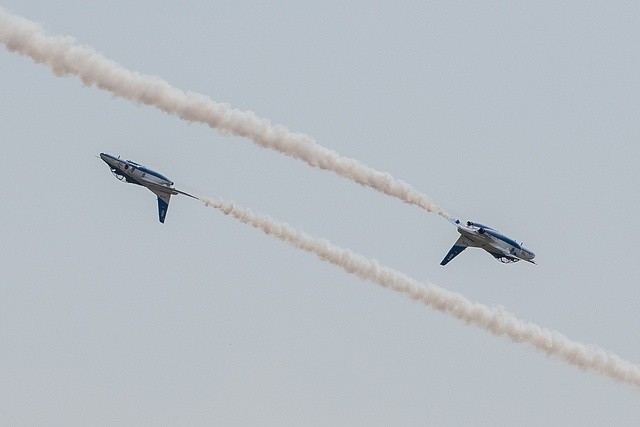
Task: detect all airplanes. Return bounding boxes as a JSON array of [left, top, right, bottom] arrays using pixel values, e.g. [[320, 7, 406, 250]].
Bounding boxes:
[[95, 151, 200, 223], [441, 213, 537, 267]]
